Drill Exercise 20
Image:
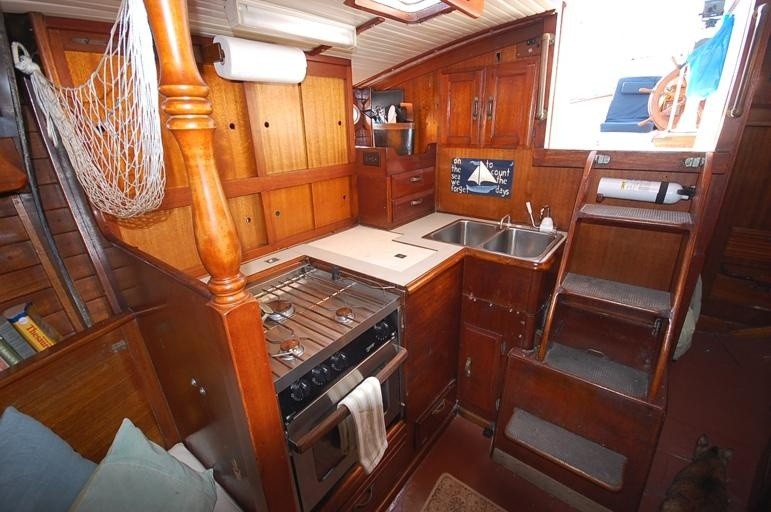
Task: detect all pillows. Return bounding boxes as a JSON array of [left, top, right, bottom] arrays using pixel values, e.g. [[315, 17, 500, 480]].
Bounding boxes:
[[0, 401, 97, 511], [62, 416, 220, 512]]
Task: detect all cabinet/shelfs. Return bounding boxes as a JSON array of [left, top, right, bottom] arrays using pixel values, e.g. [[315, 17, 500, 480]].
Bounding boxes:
[[354, 142, 441, 231], [460, 253, 559, 431], [244, 54, 357, 251], [434, 61, 539, 150], [396, 253, 465, 452], [34, 14, 206, 277], [196, 43, 273, 265]]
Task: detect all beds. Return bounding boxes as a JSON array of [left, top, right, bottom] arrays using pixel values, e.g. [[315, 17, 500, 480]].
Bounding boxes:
[[1, 306, 245, 512]]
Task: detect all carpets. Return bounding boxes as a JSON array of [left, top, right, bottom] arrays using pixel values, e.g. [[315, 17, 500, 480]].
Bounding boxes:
[[415, 471, 505, 511]]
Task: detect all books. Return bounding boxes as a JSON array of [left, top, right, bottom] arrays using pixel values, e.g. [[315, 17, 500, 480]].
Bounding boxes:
[[0, 302, 64, 370]]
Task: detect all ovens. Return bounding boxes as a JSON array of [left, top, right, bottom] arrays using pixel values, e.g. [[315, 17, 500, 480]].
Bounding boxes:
[[286, 332, 410, 512]]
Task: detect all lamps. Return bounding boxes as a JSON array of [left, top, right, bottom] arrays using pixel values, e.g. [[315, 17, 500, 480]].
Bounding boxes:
[[224, 1, 358, 50]]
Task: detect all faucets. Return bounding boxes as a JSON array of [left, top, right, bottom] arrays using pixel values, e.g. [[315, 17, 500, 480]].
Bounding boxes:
[[500, 214, 510, 231]]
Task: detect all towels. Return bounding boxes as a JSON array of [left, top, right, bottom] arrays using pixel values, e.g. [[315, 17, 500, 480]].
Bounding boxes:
[[334, 376, 389, 474]]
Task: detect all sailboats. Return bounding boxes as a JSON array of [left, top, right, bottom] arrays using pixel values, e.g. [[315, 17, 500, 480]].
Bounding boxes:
[[467, 161, 499, 194]]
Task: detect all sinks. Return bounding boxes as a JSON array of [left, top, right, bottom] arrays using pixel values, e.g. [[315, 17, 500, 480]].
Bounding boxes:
[[432, 221, 502, 247], [483, 229, 555, 258]]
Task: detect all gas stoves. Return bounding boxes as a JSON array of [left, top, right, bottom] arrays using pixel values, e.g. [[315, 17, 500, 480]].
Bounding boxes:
[[244, 260, 399, 384]]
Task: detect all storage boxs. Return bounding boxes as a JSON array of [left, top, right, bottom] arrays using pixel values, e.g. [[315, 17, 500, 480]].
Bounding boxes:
[[371, 122, 418, 158]]
